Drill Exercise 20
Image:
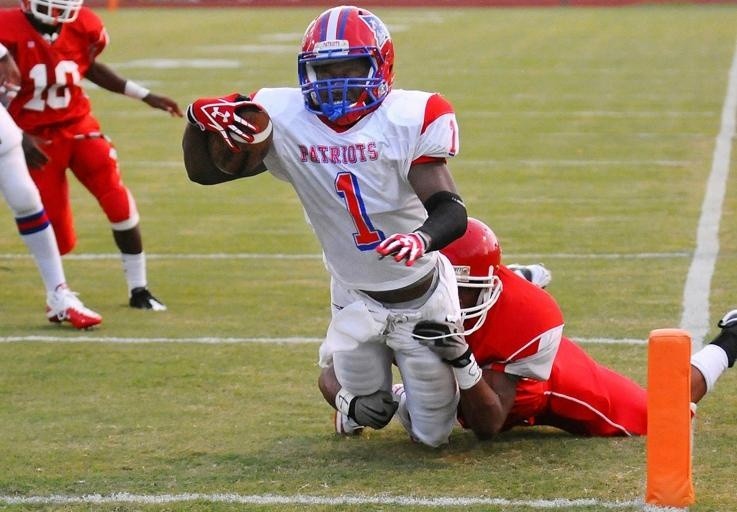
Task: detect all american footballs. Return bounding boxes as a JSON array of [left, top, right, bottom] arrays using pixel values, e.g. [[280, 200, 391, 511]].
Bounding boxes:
[[208, 105, 274, 178]]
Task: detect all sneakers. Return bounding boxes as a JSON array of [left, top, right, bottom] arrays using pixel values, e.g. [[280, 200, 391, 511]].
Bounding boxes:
[[334, 411, 364, 437], [717, 309, 737, 329], [505, 264, 552, 289], [391, 383, 421, 445], [44, 295, 103, 331], [128, 287, 167, 312]]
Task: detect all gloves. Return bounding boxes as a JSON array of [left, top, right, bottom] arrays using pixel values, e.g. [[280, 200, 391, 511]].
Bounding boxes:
[[377, 231, 426, 268], [349, 390, 399, 430], [413, 321, 472, 369], [186, 90, 261, 154]]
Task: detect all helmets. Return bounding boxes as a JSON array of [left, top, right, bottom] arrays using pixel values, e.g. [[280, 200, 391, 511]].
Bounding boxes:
[[20, 0, 85, 26], [437, 217, 502, 281], [297, 5, 395, 66]]
